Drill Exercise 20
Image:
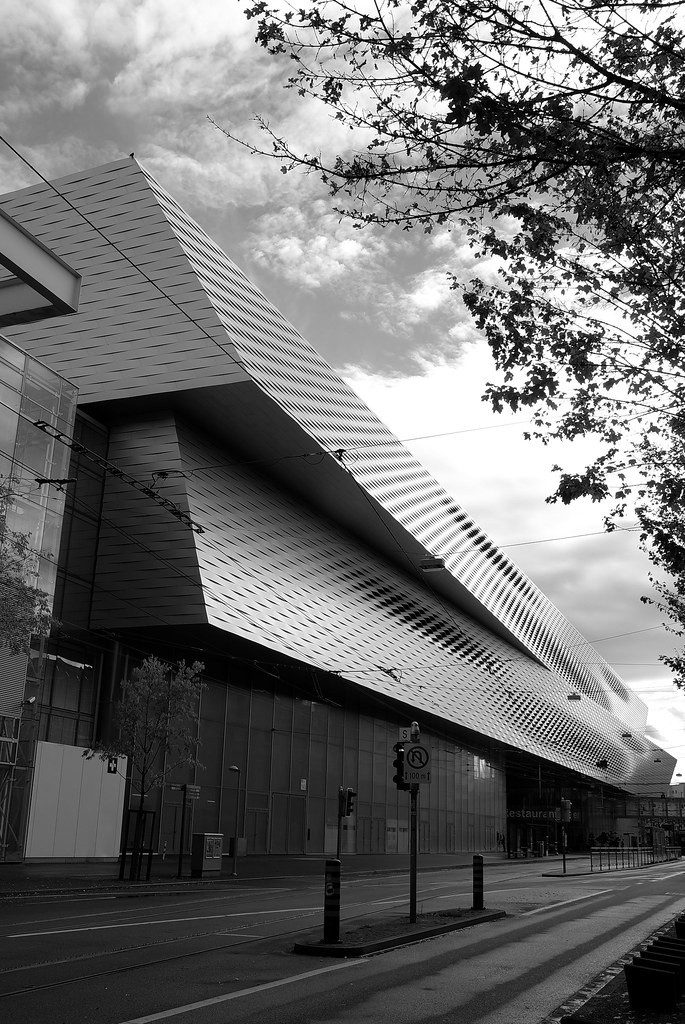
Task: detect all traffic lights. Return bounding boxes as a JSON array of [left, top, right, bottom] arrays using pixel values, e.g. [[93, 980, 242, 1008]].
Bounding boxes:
[[345, 787, 357, 818], [392, 741, 411, 791]]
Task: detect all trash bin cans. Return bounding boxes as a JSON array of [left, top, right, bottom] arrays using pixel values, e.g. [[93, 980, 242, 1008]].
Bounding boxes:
[[536, 841, 544, 857]]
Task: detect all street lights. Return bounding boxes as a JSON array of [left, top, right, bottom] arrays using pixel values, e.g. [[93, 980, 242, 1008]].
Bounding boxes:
[[228, 765, 241, 877]]
